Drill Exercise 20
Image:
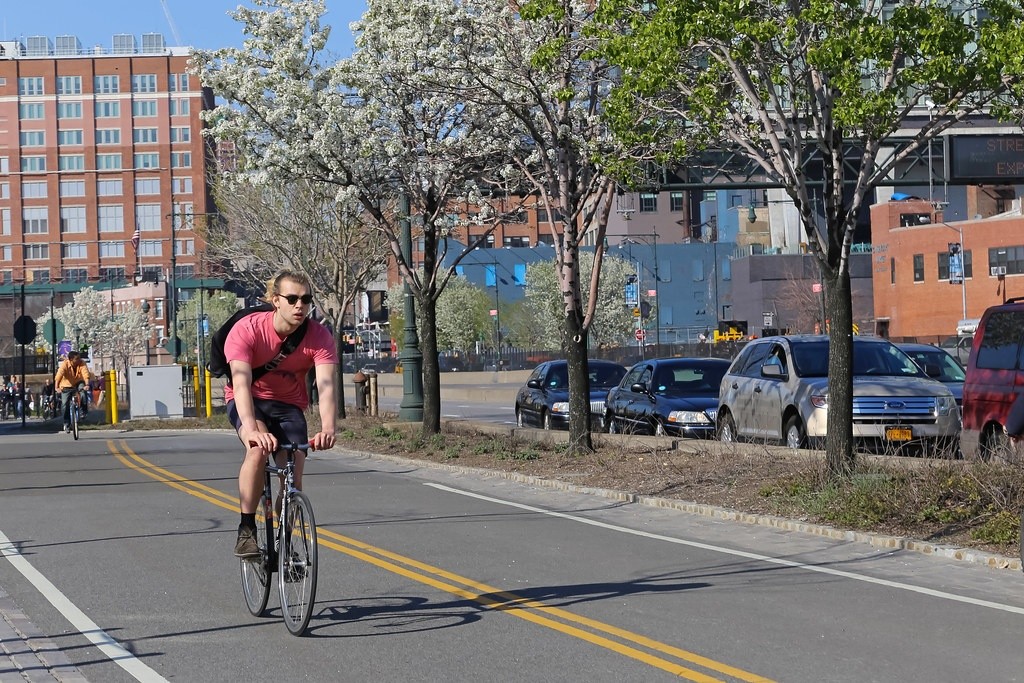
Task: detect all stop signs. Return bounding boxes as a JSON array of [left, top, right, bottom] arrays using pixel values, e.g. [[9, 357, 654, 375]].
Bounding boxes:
[[635, 330, 646, 341]]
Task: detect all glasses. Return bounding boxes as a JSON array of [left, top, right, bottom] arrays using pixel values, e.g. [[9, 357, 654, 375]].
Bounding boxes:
[[276, 293, 313, 305]]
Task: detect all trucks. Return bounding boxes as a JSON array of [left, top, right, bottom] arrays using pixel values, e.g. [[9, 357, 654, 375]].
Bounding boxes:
[[940, 319, 980, 366]]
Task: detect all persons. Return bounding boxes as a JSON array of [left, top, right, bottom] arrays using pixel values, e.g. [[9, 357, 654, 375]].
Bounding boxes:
[[81, 372, 106, 418], [224, 269, 339, 566], [0, 376, 31, 421], [55, 351, 91, 434], [40, 379, 55, 413]]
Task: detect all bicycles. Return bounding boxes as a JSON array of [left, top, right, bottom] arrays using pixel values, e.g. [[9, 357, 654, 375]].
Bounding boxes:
[[62, 388, 85, 440], [239, 439, 319, 637]]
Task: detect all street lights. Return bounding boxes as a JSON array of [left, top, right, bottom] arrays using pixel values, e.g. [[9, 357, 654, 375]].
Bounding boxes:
[[452, 256, 502, 372], [603, 225, 660, 359], [142, 211, 221, 365], [918, 217, 966, 322], [748, 188, 825, 334]]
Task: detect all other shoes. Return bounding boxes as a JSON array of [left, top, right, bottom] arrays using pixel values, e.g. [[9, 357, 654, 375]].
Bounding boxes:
[[63, 423, 71, 434], [80, 409, 88, 418]]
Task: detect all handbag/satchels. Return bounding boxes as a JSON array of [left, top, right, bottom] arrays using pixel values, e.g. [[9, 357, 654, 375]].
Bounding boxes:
[[206, 303, 309, 382]]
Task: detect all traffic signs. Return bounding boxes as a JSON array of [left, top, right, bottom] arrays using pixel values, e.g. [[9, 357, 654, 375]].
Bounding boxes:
[[764, 316, 773, 327], [762, 312, 775, 317]]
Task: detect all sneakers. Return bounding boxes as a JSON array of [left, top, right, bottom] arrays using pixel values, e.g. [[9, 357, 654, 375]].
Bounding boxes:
[[283, 552, 309, 583], [233, 522, 261, 557]]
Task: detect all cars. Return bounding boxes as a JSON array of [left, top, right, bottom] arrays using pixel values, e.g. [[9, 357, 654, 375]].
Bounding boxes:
[[519, 360, 539, 371], [439, 350, 471, 373], [515, 358, 628, 430], [714, 335, 962, 461], [395, 361, 403, 373], [483, 358, 511, 372], [892, 343, 967, 407], [361, 363, 377, 375], [602, 356, 733, 439]]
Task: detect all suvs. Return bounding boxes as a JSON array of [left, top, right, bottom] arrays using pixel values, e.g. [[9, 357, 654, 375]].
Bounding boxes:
[[960, 296, 1024, 467]]
